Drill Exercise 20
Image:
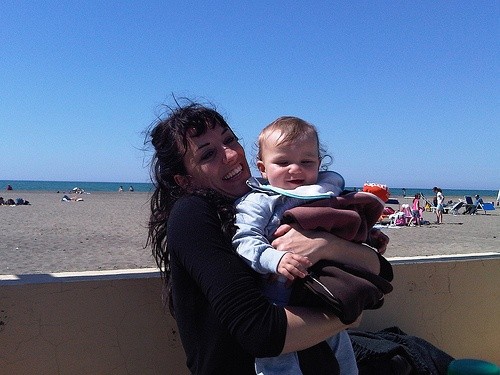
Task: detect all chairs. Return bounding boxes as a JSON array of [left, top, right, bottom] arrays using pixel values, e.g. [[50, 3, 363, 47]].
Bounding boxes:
[[464, 196, 482, 210], [444, 202, 463, 214], [420, 192, 437, 213], [475, 202, 495, 215]]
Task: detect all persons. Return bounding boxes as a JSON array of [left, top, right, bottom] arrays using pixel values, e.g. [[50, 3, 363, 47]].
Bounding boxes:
[[147, 103, 394, 375], [408, 192, 428, 227], [129, 185, 134, 192], [120, 186, 124, 192], [232, 116, 390, 375], [475, 195, 483, 204], [432, 187, 444, 224], [402, 188, 407, 197], [7, 184, 13, 190]]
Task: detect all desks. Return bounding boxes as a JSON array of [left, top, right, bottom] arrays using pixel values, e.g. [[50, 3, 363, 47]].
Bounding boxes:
[[463, 204, 475, 214]]
